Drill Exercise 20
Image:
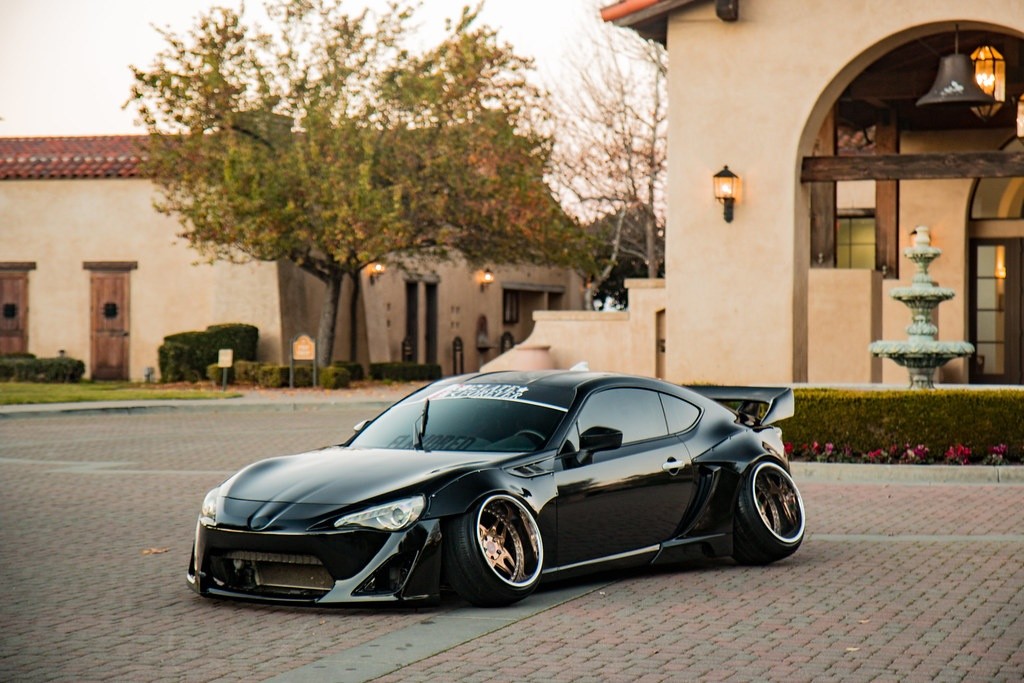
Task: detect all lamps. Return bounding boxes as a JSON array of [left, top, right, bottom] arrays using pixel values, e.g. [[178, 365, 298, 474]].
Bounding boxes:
[[968, 30, 1006, 123], [370, 261, 386, 286], [713, 164, 742, 224], [479, 267, 495, 292]]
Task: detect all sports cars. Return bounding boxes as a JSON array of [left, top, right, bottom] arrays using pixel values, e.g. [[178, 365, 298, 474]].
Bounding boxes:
[[182, 361, 807, 611]]
